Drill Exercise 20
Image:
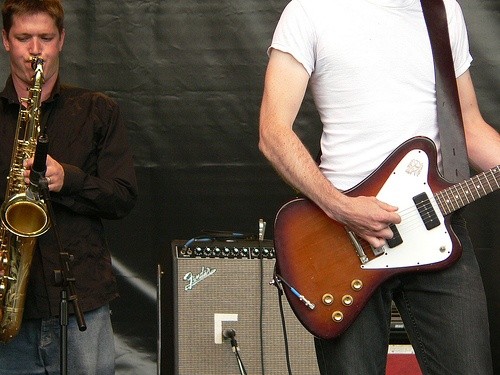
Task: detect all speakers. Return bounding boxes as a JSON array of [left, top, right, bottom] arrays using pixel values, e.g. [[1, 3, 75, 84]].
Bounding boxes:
[[172, 235, 322, 375]]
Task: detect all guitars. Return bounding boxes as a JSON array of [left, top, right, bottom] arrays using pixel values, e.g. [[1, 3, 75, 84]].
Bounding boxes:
[[272, 136, 500, 342]]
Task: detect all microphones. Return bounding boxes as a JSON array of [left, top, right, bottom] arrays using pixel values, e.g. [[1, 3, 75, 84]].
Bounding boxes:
[[25, 138, 49, 201], [223, 328, 235, 337]]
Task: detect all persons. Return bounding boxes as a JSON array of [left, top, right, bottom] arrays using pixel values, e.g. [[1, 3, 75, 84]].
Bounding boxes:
[[256, 0, 500, 375], [0, 0, 138, 375]]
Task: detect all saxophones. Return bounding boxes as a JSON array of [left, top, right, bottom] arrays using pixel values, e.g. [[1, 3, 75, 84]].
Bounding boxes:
[[0, 56, 53, 344]]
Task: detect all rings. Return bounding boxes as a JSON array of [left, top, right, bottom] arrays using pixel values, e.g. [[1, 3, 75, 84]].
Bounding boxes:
[[46, 176, 51, 185]]
